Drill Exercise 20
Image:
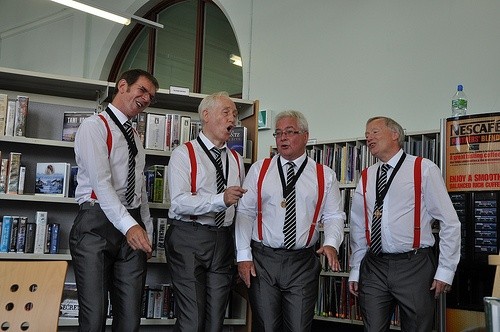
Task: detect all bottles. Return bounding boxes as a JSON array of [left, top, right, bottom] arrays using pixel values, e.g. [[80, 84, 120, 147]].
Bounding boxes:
[[452, 85, 467, 117]]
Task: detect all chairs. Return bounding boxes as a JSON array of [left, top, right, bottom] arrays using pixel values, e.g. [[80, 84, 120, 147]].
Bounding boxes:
[[0, 253, 73, 332]]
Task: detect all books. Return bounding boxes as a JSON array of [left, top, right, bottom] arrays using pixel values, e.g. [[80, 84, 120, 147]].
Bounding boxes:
[[306, 136, 437, 326], [1, 93, 253, 317]]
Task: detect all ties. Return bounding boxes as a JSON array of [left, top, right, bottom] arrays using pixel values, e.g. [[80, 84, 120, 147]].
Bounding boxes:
[[283, 161, 296, 250], [370, 164, 392, 257], [212, 147, 226, 229], [123, 121, 135, 206]]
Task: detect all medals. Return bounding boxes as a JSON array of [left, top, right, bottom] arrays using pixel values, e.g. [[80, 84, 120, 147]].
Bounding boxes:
[[374, 210, 381, 218], [281, 200, 286, 208]]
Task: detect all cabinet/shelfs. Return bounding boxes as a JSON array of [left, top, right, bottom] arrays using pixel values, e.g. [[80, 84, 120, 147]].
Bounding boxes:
[[0, 66, 260, 332], [269, 128, 441, 332]]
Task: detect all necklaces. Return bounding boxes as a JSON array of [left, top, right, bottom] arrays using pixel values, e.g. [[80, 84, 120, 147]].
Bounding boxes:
[[104, 105, 139, 156], [196, 133, 229, 192]]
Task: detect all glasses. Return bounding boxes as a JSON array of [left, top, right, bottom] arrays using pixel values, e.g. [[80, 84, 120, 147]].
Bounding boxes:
[[273, 130, 307, 138]]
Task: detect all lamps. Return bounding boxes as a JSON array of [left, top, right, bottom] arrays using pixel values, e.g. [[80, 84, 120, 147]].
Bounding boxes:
[[50, 0, 165, 30], [226, 53, 242, 67]]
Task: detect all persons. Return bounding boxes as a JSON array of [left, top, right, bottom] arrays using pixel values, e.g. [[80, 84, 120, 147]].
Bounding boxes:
[[165, 92, 248, 332], [68, 69, 159, 332], [236, 110, 346, 332], [347, 116, 460, 332]]
